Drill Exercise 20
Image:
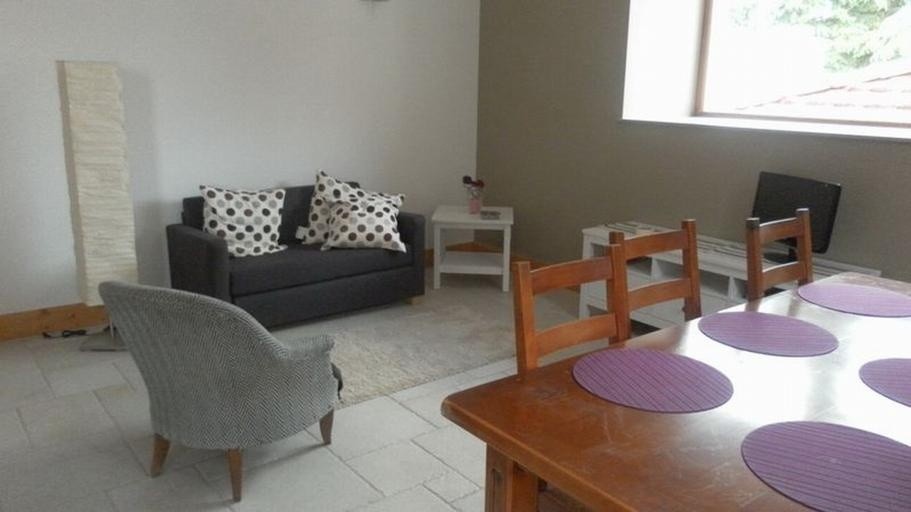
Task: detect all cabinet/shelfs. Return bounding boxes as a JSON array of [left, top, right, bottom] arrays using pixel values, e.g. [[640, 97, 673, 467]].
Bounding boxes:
[[578, 217, 884, 331]]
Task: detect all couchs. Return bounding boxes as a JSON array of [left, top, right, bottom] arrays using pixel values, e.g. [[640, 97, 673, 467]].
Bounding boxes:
[[165, 182, 424, 332]]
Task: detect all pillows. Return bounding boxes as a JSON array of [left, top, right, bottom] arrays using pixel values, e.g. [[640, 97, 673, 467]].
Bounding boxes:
[[200, 185, 289, 257], [296, 172, 359, 248], [321, 189, 406, 253]]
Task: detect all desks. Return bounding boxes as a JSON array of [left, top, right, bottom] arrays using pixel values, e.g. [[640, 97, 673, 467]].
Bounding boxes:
[[442, 272, 911, 512]]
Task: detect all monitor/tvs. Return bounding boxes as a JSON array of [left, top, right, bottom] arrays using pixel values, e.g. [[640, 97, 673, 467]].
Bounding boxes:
[[750, 171, 841, 264]]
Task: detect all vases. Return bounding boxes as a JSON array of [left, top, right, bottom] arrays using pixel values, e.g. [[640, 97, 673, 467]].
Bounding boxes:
[[466, 198, 483, 215]]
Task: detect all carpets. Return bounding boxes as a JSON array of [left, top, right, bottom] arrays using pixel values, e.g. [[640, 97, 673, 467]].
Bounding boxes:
[[266, 260, 606, 410]]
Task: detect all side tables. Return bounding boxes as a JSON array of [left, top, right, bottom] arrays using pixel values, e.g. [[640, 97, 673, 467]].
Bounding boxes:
[[432, 205, 515, 292]]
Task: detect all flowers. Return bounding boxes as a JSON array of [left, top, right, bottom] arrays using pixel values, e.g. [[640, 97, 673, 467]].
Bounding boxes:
[[461, 172, 486, 198]]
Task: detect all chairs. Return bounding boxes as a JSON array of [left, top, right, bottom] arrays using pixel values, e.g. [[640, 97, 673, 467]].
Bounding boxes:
[[606, 219, 701, 339], [514, 243, 631, 512], [744, 209, 814, 303], [98, 282, 343, 503]]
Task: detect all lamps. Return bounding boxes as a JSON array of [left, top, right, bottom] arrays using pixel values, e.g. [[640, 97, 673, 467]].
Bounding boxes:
[[55, 60, 139, 352]]
